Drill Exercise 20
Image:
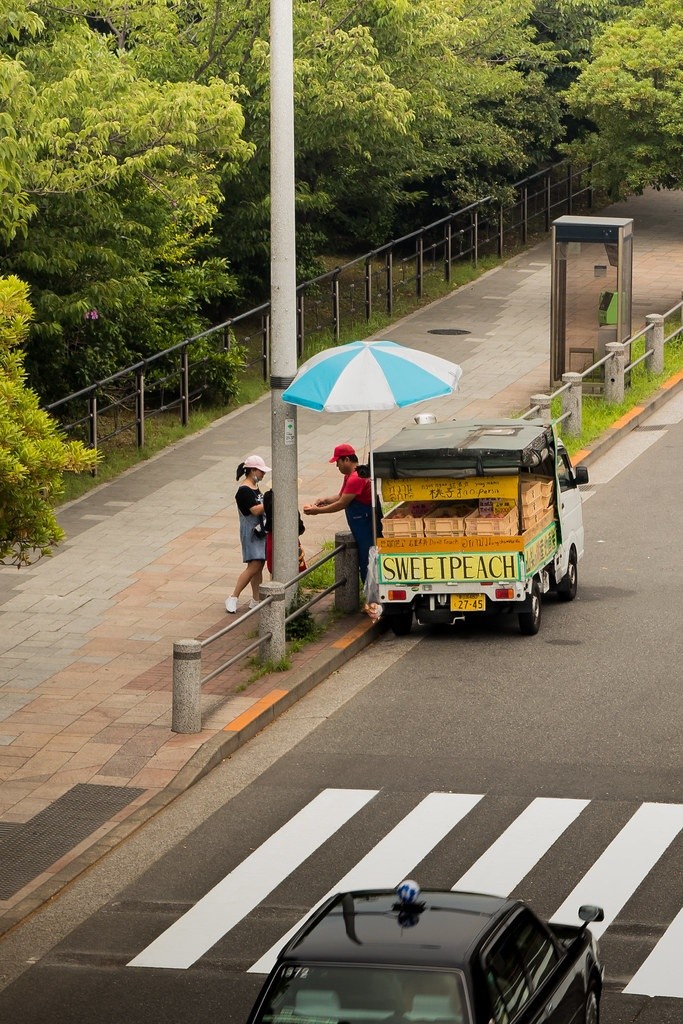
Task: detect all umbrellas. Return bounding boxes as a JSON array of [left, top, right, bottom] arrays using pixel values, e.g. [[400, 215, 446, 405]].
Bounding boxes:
[[283, 342, 461, 544]]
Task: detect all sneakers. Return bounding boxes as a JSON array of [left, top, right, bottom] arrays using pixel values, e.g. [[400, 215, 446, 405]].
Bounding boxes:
[[225, 594, 239, 613], [249, 599, 262, 608]]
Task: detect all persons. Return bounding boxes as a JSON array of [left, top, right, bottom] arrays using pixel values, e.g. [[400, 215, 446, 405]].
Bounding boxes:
[[304, 444, 385, 583], [225, 455, 305, 614]]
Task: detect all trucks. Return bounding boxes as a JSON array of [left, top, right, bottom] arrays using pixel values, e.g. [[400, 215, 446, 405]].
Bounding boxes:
[[351, 416, 588, 636]]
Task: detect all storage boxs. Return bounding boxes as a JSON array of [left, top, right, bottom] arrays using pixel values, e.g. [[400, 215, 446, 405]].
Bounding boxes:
[[381, 472, 554, 538]]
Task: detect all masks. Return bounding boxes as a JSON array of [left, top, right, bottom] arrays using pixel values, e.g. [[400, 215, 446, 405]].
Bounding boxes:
[[253, 474, 259, 483]]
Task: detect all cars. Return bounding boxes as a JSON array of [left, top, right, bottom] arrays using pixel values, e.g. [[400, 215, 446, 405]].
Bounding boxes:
[[246, 880, 605, 1024]]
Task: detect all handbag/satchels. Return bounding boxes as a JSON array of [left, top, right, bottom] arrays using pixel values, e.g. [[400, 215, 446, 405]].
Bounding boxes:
[[251, 493, 268, 543], [362, 546, 384, 624]]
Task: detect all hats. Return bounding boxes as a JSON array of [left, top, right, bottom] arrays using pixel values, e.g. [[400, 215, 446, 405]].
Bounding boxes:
[[243, 455, 272, 472], [329, 444, 356, 463]]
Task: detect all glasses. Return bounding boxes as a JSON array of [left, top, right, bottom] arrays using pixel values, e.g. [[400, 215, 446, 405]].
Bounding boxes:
[[258, 469, 265, 475]]
